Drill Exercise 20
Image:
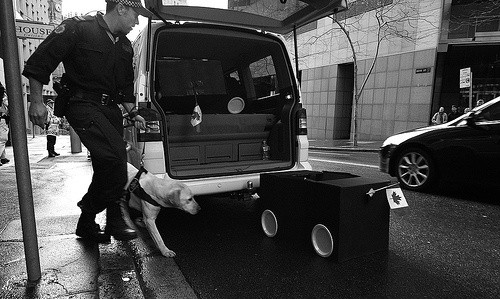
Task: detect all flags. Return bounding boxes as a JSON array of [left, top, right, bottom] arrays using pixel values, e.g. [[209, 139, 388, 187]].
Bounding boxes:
[[191, 105, 203, 127], [385, 188, 409, 209]]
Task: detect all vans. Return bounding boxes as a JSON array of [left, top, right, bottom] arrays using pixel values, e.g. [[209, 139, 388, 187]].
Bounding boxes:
[[129, 0, 350, 198]]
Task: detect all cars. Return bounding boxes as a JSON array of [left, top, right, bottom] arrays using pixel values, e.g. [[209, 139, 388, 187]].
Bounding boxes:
[[378, 95, 500, 191]]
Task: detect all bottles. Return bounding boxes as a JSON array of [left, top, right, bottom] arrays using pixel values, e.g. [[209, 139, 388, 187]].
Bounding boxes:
[[261, 141, 270, 160], [244, 181, 257, 201]]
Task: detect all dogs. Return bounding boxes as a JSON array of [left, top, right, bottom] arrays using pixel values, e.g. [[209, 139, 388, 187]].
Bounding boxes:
[[124, 161, 201, 257]]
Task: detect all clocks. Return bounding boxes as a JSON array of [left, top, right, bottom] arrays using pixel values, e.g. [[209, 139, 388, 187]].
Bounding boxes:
[[54, 3, 62, 13]]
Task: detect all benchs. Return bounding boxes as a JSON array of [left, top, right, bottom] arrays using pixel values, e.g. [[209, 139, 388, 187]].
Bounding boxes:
[[166, 112, 276, 167], [157, 58, 227, 95]]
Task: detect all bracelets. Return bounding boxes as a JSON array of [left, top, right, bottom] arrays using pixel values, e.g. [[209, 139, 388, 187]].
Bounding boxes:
[[129, 110, 139, 119]]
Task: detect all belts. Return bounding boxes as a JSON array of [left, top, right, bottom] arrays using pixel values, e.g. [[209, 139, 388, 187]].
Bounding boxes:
[[72, 86, 110, 106]]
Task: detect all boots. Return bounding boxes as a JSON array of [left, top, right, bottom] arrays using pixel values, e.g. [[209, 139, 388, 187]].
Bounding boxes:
[[105, 204, 137, 240], [76, 212, 110, 241]]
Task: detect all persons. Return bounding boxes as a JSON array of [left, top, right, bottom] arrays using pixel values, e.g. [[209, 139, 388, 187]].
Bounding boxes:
[[0, 81, 10, 166], [46, 99, 60, 158], [432, 107, 448, 126], [21, 0, 153, 243], [448, 100, 484, 122]]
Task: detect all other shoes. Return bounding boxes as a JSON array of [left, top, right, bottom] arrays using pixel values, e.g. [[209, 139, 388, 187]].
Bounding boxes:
[[1, 158, 9, 164], [49, 151, 60, 157]]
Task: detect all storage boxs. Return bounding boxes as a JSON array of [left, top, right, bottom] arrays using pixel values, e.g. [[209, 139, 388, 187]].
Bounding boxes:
[[261, 168, 391, 265]]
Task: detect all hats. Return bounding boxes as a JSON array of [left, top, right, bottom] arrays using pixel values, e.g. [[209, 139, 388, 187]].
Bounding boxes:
[[105, 0, 154, 17], [439, 107, 444, 112], [45, 99, 54, 104]]
